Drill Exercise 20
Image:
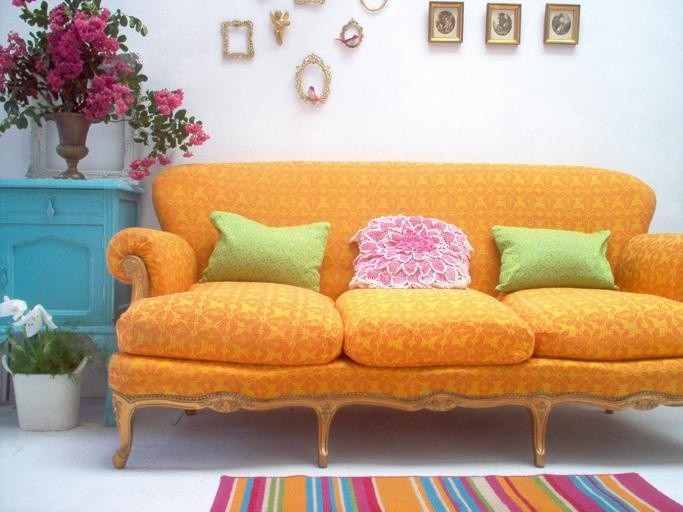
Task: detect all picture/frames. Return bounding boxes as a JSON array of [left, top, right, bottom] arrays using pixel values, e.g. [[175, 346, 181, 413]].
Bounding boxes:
[[543, 4, 581, 46], [427, 1, 465, 44], [484, 3, 521, 45]]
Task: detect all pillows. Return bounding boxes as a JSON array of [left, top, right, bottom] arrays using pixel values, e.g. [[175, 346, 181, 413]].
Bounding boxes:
[[487, 222, 619, 291], [347, 213, 473, 291], [202, 209, 329, 294]]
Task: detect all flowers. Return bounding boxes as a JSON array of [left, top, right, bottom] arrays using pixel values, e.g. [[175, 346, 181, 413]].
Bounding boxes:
[[0, 2, 206, 181], [0, 296, 88, 378]]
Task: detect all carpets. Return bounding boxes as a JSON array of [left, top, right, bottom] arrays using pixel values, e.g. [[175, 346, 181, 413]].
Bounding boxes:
[[203, 471, 683, 511]]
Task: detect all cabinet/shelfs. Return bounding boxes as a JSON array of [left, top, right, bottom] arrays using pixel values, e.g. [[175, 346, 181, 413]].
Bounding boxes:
[[1, 180, 140, 431]]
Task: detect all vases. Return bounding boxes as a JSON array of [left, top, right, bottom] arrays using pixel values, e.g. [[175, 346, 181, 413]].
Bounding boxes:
[[41, 110, 90, 180], [0, 354, 90, 433]]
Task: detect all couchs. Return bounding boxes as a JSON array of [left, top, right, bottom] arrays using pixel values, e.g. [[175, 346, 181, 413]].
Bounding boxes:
[[105, 160, 681, 470]]
